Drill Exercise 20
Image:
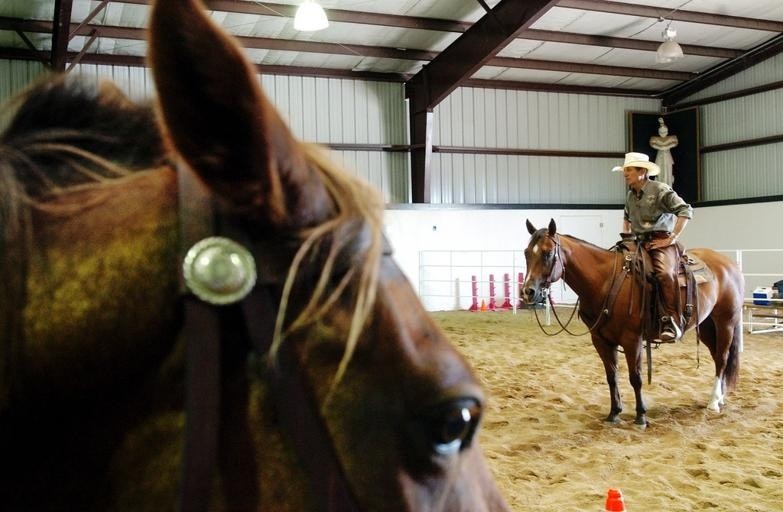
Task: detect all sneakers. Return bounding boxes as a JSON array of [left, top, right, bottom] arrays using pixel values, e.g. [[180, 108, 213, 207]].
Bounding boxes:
[[661, 330, 675, 340]]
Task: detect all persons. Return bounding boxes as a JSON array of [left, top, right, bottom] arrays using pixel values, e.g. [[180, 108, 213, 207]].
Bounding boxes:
[[611, 151, 695, 343]]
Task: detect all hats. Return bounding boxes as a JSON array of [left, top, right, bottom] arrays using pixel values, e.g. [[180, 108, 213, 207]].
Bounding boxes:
[[612, 152, 660, 176]]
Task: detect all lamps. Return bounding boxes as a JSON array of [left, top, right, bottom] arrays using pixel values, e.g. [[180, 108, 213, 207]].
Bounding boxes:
[[293, 0, 330, 31], [656, 14, 685, 63]]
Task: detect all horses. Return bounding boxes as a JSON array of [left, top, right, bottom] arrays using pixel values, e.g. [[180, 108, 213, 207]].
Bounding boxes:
[[521, 219, 745, 431], [0, 0, 512, 512]]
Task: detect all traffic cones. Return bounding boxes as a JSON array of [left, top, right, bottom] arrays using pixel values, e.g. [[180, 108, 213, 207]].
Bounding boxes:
[[480, 299, 489, 313]]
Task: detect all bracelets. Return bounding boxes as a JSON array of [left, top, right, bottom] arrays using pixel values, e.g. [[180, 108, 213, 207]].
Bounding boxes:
[[668, 231, 678, 241]]
